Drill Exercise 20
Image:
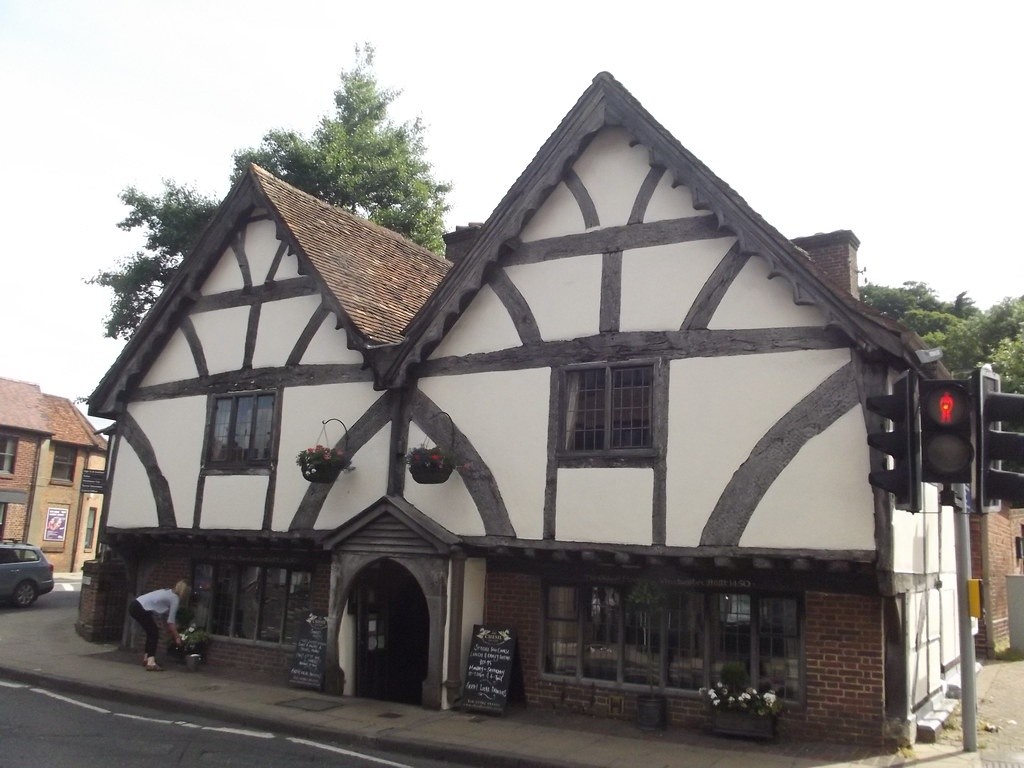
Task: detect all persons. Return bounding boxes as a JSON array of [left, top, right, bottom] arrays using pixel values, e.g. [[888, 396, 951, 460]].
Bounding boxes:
[[130, 579, 192, 671]]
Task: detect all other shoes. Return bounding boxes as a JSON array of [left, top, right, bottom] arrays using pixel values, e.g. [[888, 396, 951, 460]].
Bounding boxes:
[[143, 661, 147, 666], [146, 664, 166, 672]]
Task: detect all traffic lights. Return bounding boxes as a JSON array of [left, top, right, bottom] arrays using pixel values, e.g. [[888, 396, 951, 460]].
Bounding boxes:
[[917, 376, 973, 484], [864, 367, 914, 511], [975, 366, 1024, 513]]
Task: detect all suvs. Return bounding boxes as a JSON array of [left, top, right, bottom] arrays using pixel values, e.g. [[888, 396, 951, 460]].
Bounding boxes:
[[0, 538, 55, 610]]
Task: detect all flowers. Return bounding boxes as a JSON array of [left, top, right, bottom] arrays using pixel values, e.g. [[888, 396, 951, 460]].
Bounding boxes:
[[173, 608, 209, 649], [401, 446, 474, 471], [295, 445, 357, 477], [699, 662, 784, 717]]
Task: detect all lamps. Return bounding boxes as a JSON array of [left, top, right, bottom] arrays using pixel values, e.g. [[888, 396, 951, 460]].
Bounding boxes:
[[916, 347, 943, 369]]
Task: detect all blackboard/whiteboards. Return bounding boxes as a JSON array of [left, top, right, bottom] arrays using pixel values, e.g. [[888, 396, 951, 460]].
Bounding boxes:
[[460, 624, 518, 715], [287, 609, 328, 689]]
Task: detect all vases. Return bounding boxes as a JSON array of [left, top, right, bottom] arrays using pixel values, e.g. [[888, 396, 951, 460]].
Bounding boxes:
[[409, 467, 452, 484], [164, 643, 207, 663], [300, 464, 340, 483], [712, 707, 774, 740]]
